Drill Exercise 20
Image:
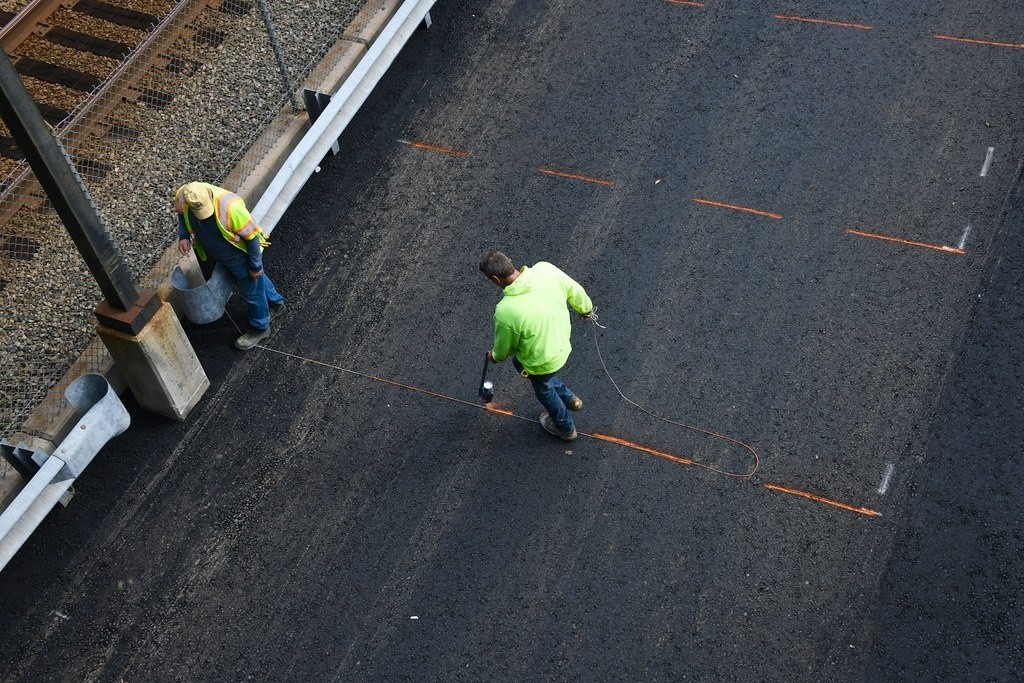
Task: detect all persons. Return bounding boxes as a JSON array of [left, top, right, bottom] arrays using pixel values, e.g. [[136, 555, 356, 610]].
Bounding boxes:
[[479, 249, 594, 441], [175, 181, 286, 351]]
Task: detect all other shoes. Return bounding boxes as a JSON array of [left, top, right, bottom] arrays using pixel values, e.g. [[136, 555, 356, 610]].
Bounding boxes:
[[268, 302, 286, 322], [566, 396, 583, 410], [235, 325, 272, 351], [540, 412, 577, 441]]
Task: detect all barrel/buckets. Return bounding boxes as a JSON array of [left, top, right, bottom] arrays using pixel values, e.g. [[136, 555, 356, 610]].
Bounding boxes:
[[172, 262, 225, 324]]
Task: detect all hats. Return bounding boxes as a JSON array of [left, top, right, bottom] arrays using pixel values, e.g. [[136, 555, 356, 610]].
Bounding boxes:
[[184, 181, 214, 220]]
